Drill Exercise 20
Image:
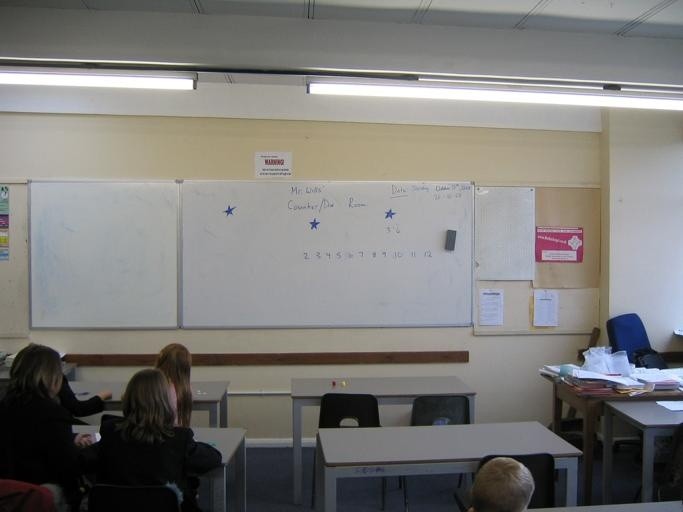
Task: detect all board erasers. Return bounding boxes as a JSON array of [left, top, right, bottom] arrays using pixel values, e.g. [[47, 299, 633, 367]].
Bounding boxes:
[[445, 230, 456, 251]]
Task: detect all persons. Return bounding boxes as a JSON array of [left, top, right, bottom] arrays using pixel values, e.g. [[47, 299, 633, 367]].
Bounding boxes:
[[78, 369, 222, 512], [634, 422, 683, 502], [154, 343, 193, 428], [469, 457, 535, 511], [0, 343, 96, 511], [55, 374, 113, 417]]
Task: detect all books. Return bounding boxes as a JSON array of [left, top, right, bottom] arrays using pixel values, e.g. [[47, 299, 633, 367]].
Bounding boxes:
[[539, 363, 680, 399]]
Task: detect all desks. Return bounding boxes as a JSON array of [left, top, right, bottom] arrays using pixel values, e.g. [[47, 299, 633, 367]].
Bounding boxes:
[[0, 368, 683, 512]]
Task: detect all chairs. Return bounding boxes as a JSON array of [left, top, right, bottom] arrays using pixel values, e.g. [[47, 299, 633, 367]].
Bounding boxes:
[[606, 313, 670, 368]]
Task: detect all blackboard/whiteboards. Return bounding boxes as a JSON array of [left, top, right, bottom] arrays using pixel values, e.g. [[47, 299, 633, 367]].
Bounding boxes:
[[179, 182, 473, 328], [24, 177, 180, 331]]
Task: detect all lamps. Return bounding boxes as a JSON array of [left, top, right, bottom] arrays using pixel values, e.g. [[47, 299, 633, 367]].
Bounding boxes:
[[305, 78, 683, 113], [0, 65, 199, 91]]
